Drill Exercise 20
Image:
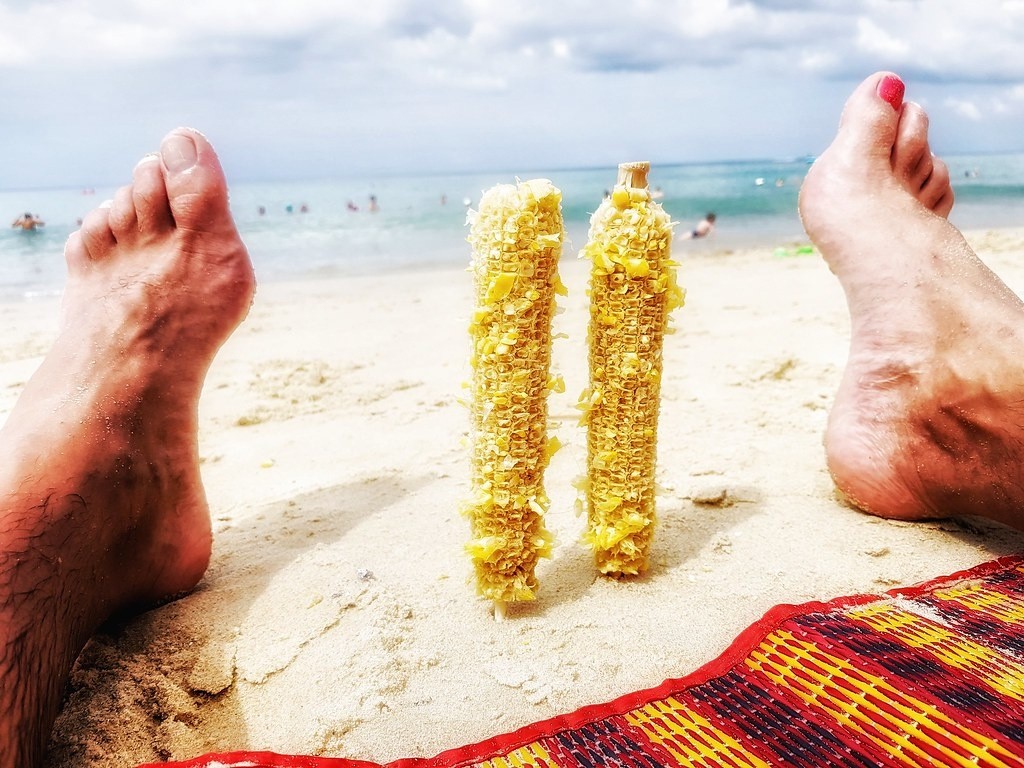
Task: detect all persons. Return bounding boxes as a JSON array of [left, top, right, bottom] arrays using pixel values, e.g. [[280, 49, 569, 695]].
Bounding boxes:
[[802, 77, 1024, 528], [605, 177, 784, 199], [2, 130, 256, 768], [259, 194, 448, 215], [682, 211, 716, 240], [12, 211, 45, 232]]
